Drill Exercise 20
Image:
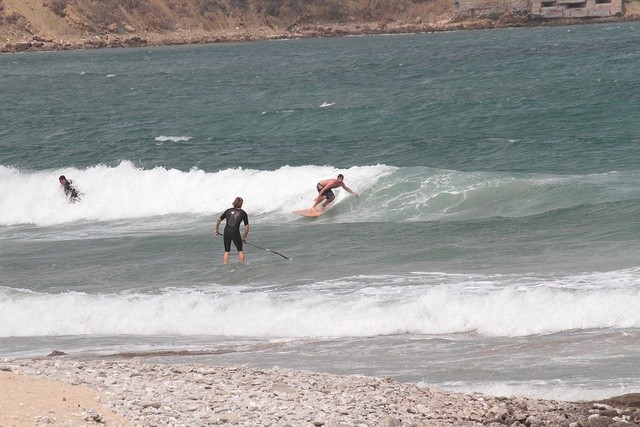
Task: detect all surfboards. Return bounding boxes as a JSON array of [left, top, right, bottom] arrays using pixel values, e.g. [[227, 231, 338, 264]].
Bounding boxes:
[[293, 205, 333, 217]]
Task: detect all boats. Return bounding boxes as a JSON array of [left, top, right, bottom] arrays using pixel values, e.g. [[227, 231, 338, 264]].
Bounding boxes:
[[293, 205, 337, 219]]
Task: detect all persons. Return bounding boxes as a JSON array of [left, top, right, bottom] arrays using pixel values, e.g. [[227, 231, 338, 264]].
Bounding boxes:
[[59, 175, 78, 202], [311, 173, 358, 212], [215, 196, 249, 264]]
